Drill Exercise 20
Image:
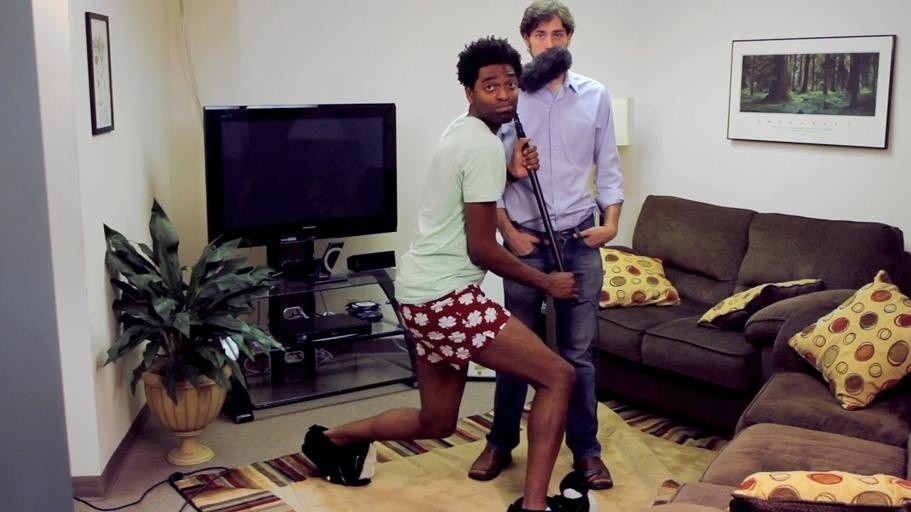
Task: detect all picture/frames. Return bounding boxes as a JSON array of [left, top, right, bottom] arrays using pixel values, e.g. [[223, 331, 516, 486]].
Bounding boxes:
[[727, 35, 895, 149], [84, 10, 115, 137]]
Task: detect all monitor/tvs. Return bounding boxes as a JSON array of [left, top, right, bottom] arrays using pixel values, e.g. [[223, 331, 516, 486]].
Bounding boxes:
[[205, 103, 398, 244]]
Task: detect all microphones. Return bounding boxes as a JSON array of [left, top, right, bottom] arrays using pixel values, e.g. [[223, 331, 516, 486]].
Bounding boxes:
[[518, 47, 571, 94]]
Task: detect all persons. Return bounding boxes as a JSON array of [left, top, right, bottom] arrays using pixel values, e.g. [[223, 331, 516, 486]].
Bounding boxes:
[[468, 0, 613, 489], [301, 37, 578, 512]]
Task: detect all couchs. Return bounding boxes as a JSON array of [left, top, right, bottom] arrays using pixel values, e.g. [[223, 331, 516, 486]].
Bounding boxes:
[[654, 286, 911, 512], [548, 194, 911, 433]]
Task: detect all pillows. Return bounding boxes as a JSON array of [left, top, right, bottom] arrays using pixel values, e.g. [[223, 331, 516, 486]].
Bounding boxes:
[[596, 246, 682, 308], [788, 269, 911, 410], [731, 468, 911, 512], [697, 277, 824, 330]]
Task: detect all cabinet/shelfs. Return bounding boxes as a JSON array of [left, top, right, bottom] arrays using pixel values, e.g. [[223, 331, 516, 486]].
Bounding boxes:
[[221, 268, 417, 425]]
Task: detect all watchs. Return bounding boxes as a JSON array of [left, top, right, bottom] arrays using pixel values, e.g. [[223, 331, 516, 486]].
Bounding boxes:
[[507, 165, 519, 182]]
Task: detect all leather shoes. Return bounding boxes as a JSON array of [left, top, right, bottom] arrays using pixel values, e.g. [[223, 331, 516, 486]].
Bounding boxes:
[[468, 443, 513, 480], [573, 456, 613, 490]]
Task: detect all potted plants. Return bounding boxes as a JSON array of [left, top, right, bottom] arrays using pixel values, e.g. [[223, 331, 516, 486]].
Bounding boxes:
[[96, 199, 285, 467]]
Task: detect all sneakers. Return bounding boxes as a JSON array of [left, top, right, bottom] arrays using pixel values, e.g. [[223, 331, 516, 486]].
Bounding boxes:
[[508, 493, 597, 512], [300, 425, 373, 487]]
